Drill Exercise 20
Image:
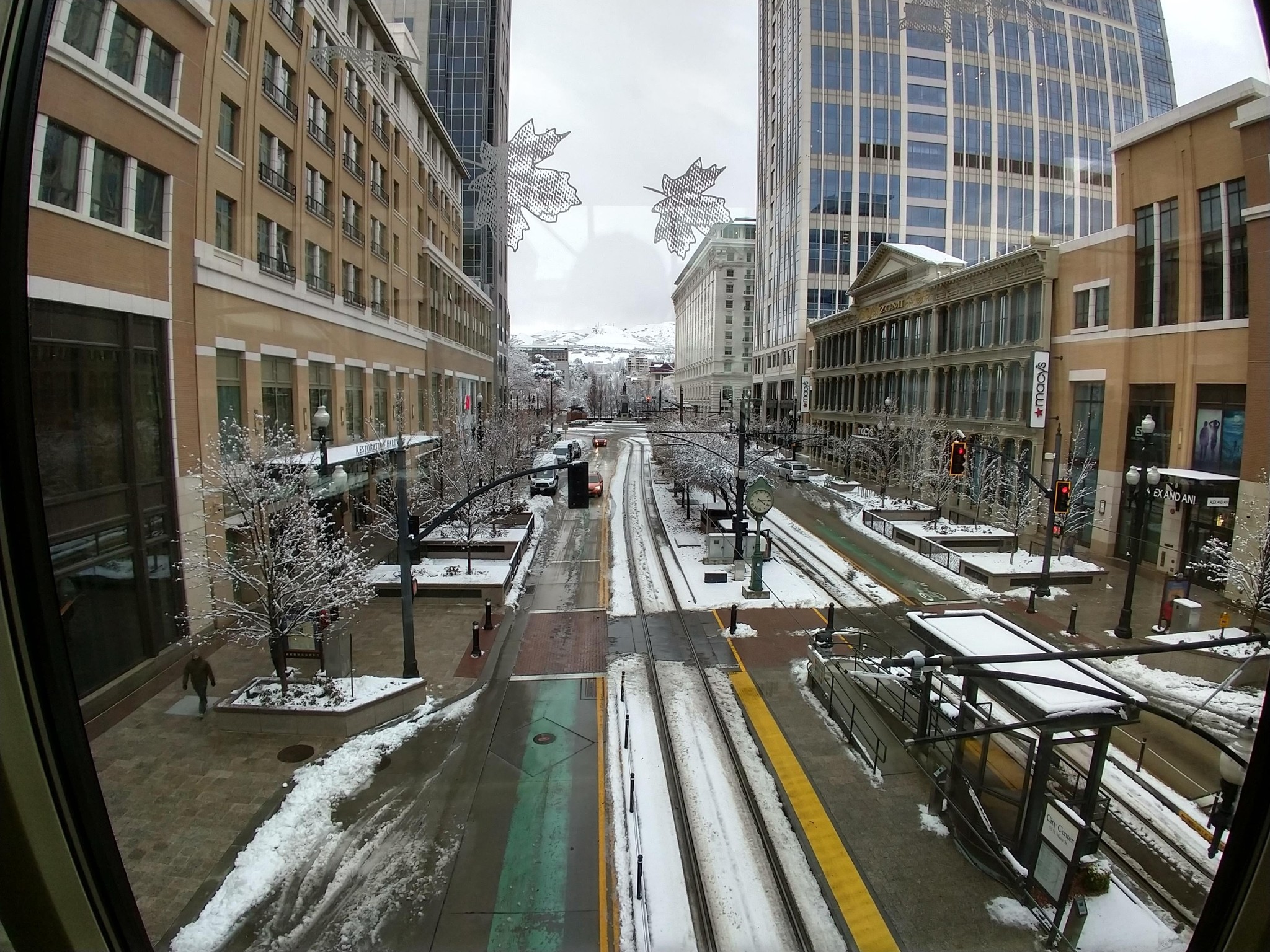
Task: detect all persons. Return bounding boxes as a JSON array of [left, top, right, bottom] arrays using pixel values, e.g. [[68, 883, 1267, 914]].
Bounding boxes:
[[1197, 419, 1209, 465], [182, 649, 216, 719], [1209, 419, 1220, 465]]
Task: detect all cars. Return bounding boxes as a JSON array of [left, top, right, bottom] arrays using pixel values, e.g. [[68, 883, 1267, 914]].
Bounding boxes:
[[592, 435, 608, 447], [589, 471, 604, 497], [573, 442, 581, 458]]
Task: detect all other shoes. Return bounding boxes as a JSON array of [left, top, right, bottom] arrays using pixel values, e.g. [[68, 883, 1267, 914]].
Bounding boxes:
[[199, 713, 206, 718]]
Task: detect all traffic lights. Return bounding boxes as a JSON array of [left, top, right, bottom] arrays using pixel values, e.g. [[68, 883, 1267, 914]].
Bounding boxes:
[[647, 397, 650, 403], [1054, 481, 1070, 514], [950, 442, 966, 475], [1052, 524, 1063, 536]]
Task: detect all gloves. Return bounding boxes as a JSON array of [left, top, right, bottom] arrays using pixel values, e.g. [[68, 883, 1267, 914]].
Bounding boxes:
[[211, 680, 216, 686], [183, 685, 187, 690]]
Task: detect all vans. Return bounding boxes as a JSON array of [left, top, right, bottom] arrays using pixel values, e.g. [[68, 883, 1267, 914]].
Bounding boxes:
[[550, 441, 574, 464], [528, 454, 559, 496]]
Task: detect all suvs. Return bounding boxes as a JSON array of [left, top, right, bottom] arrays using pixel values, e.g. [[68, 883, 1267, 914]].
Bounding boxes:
[[568, 419, 588, 428], [777, 461, 809, 482]]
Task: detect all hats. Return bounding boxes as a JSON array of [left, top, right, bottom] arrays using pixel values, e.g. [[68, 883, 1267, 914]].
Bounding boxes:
[[192, 648, 199, 656]]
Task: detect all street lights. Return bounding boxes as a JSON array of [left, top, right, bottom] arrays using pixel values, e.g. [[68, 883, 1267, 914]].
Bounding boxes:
[[789, 392, 802, 460], [302, 406, 348, 623], [877, 396, 895, 496], [733, 466, 748, 564], [470, 391, 490, 488], [1114, 414, 1161, 639], [745, 390, 754, 450]]
